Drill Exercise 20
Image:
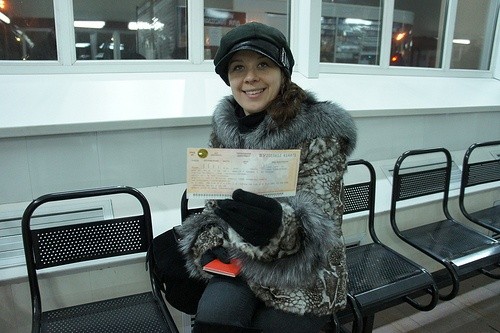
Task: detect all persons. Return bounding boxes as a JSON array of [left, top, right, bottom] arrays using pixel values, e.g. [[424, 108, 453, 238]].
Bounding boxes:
[[145, 22, 358, 332]]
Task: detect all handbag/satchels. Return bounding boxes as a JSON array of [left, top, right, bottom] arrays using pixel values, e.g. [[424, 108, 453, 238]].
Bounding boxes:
[[145, 225, 208, 315]]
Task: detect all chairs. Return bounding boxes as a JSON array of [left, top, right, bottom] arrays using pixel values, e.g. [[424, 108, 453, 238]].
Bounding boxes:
[[459, 140, 500, 235], [343, 160, 439, 333], [390, 147, 500, 302], [21, 186, 180, 333]]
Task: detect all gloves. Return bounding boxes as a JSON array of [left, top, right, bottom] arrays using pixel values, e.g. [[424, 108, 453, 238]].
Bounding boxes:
[[200, 246, 230, 279], [214, 188, 282, 247]]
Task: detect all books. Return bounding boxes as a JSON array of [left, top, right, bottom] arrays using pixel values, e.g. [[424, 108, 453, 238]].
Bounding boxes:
[[203, 256, 244, 278]]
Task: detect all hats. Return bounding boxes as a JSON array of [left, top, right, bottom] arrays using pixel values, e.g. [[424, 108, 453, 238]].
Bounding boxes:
[[214, 21, 294, 86]]
